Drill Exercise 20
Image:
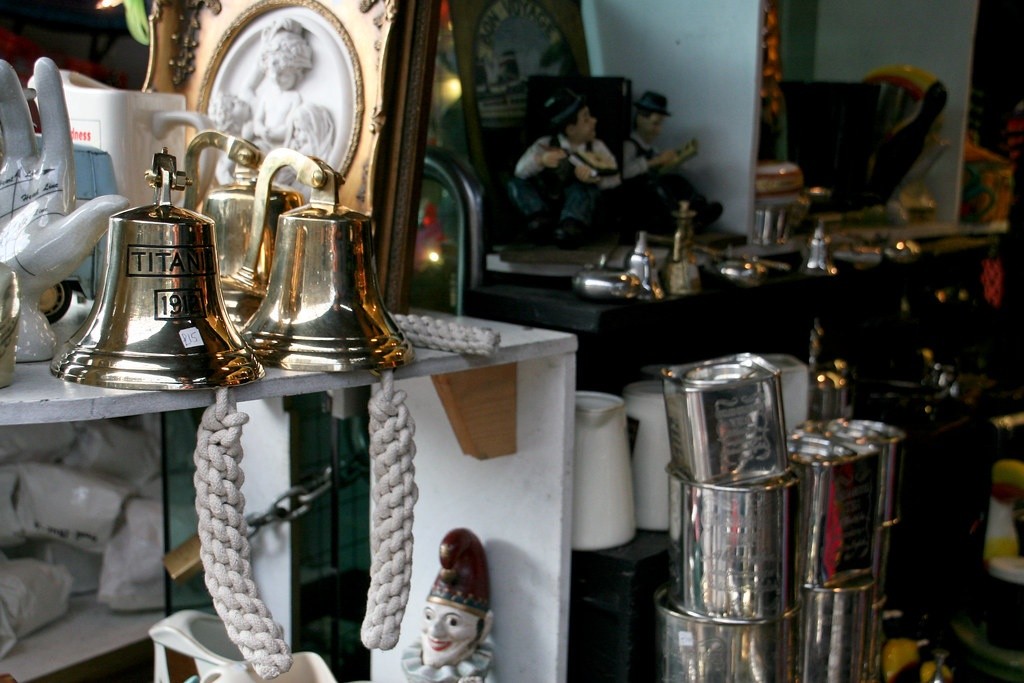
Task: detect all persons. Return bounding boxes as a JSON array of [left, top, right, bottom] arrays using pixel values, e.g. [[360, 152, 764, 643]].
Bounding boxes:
[[400, 528, 495, 683], [622, 92, 723, 235], [509, 87, 617, 248], [212, 19, 335, 161]]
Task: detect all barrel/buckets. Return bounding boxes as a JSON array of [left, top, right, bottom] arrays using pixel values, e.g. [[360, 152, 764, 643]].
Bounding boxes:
[[653, 352, 908, 683], [626, 382, 672, 531]]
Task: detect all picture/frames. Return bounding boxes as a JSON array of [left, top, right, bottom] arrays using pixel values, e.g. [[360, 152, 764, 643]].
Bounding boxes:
[[150, 0, 443, 314]]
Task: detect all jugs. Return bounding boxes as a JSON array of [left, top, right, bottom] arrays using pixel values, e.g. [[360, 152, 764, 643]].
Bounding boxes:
[[27, 69, 216, 209], [569, 391, 636, 550]]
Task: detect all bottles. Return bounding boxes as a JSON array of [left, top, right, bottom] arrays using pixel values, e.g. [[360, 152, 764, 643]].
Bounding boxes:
[[921, 366, 985, 437], [753, 162, 811, 247]]
